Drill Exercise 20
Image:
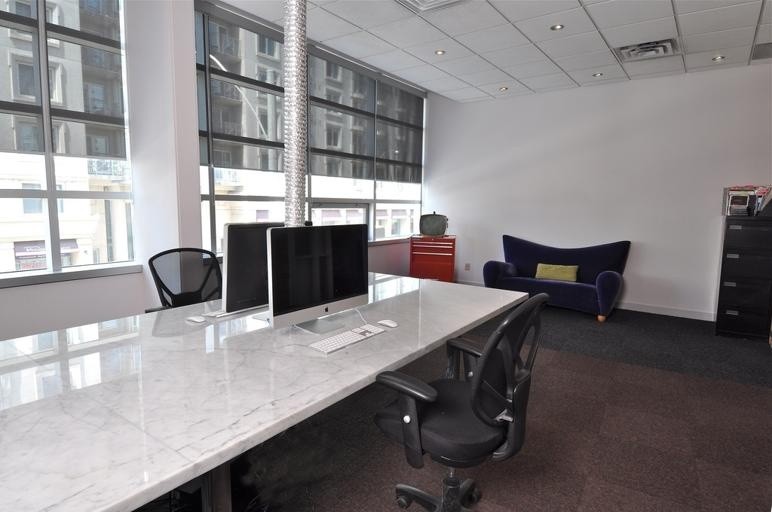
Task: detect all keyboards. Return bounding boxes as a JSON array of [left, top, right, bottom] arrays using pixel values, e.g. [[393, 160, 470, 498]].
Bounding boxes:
[[309, 323, 385, 354]]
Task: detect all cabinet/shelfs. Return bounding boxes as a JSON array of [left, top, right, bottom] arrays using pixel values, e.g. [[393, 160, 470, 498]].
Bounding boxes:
[[714, 215, 772, 339], [408, 233, 456, 282]]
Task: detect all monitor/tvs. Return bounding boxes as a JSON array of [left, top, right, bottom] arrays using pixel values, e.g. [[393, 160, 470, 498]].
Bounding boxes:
[[221, 221, 284, 315], [266, 224, 369, 335]]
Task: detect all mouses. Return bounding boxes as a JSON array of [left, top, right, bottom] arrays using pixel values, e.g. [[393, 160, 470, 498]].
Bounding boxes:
[[187, 316, 205, 323], [379, 319, 398, 328]]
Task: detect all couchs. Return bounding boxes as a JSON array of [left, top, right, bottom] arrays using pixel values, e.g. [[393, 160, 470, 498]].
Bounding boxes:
[[482, 234, 631, 322]]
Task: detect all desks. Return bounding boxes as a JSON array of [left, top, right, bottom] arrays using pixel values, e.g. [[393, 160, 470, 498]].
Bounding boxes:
[[0, 269, 530, 512]]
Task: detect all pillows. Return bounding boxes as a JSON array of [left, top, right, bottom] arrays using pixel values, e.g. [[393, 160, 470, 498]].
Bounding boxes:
[[535, 263, 579, 282]]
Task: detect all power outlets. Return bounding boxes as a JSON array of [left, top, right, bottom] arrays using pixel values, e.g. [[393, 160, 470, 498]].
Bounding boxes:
[[464, 263, 472, 271]]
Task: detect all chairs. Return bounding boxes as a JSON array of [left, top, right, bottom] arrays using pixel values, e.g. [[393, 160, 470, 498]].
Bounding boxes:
[[375, 293, 549, 511], [145, 247, 222, 313]]
